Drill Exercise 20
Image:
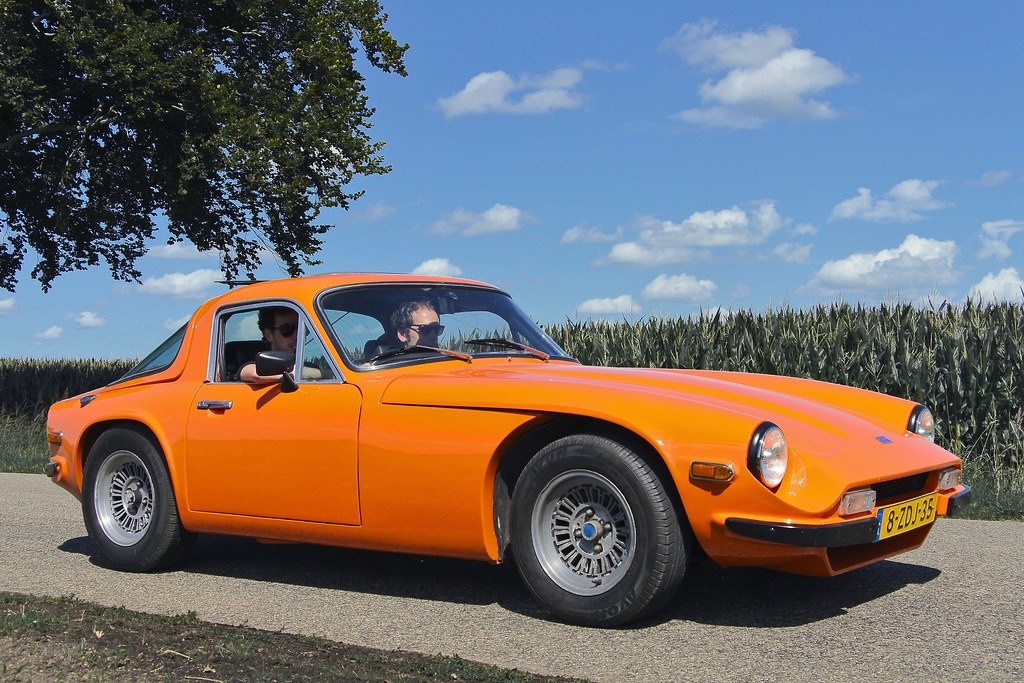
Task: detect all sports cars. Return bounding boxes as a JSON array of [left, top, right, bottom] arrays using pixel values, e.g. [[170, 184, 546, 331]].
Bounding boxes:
[[46, 274, 973, 627]]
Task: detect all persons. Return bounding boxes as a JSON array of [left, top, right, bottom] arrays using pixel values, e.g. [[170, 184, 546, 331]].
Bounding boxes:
[[389, 300, 445, 353], [237, 306, 332, 383]]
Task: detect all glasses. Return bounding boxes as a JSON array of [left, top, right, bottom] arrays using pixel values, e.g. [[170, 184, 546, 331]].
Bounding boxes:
[[267, 322, 298, 337], [408, 324, 445, 336]]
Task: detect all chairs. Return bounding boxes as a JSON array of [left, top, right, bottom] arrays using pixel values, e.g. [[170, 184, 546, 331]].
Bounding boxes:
[[224, 340, 262, 384], [364, 340, 383, 362]]
